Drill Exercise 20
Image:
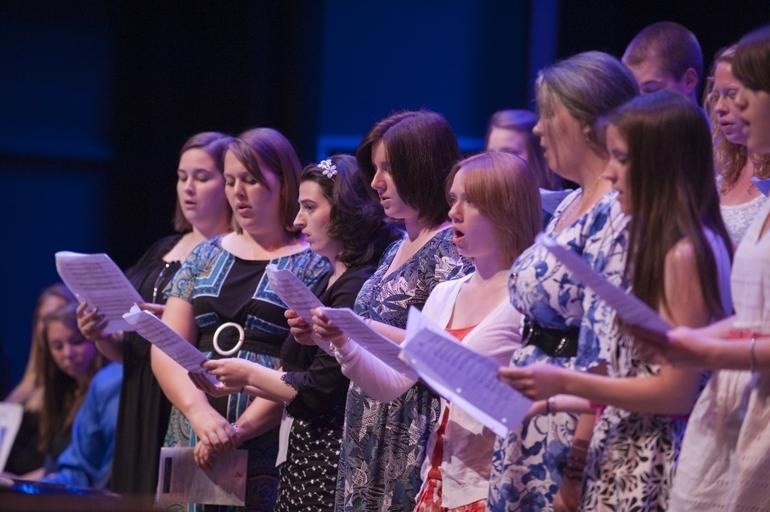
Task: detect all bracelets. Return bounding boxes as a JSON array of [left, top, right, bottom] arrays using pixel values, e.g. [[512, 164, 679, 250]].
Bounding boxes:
[[747, 336, 757, 372], [563, 443, 589, 483], [545, 396, 557, 417]]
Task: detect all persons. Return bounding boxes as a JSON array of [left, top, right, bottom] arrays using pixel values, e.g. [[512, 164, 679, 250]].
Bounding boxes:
[[622, 21, 700, 106], [487, 52, 644, 509], [283, 111, 477, 509], [614, 29, 770, 511], [500, 88, 736, 512], [309, 152, 542, 511], [40, 304, 100, 471], [190, 154, 383, 512], [151, 128, 309, 512], [0, 285, 77, 476], [713, 41, 768, 248], [488, 109, 555, 225], [20, 363, 123, 492], [76, 132, 237, 498]]
